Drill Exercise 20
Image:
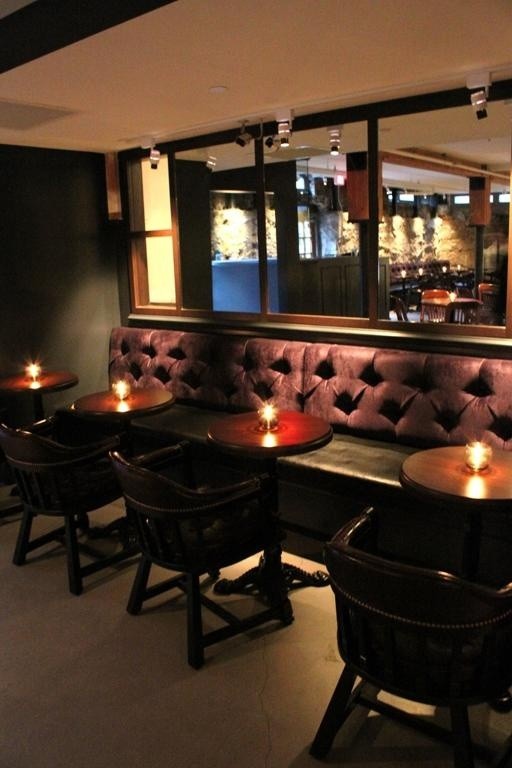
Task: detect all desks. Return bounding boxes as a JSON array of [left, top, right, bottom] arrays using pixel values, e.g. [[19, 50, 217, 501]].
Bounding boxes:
[[68, 389, 176, 536], [0, 370, 78, 498], [398, 444, 512, 585], [208, 411, 333, 623], [420, 298, 484, 322]]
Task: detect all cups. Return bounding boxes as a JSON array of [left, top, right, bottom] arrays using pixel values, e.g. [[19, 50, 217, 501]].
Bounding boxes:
[[256, 402, 281, 426], [462, 441, 494, 470], [111, 379, 131, 400]]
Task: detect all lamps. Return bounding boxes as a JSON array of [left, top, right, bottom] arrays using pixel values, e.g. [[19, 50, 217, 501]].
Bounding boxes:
[[465, 72, 492, 120], [265, 136, 275, 149], [140, 138, 161, 170], [327, 124, 343, 156], [235, 118, 264, 146], [275, 109, 294, 147]]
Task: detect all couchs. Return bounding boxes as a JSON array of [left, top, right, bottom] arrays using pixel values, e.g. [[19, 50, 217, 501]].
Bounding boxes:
[[108, 327, 512, 505], [390, 260, 450, 305]]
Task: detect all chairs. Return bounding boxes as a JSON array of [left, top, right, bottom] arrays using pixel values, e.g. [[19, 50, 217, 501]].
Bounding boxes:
[[307, 504, 511, 767], [478, 283, 497, 323], [106, 441, 294, 668], [389, 295, 413, 323], [444, 301, 482, 324], [0, 416, 142, 593], [421, 289, 451, 323]]
